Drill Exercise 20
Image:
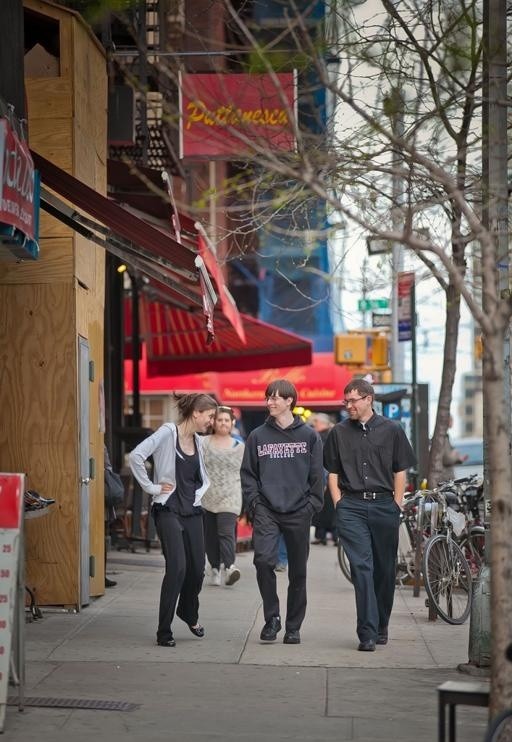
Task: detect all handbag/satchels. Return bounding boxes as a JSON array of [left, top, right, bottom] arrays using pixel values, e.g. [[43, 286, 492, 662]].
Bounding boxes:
[[103, 469, 125, 507]]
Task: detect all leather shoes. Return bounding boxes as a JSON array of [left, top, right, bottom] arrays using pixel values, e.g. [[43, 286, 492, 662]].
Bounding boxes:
[[358, 639, 375, 653], [377, 633, 389, 644], [157, 637, 176, 646], [283, 635, 301, 645], [260, 618, 281, 641], [187, 622, 204, 638], [105, 578, 116, 589]]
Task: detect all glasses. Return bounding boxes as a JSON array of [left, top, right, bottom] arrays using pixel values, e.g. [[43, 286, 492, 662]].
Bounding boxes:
[[264, 397, 285, 402], [343, 395, 367, 405]]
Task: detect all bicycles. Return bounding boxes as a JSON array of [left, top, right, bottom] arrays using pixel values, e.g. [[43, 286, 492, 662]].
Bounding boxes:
[[338, 473, 485, 624]]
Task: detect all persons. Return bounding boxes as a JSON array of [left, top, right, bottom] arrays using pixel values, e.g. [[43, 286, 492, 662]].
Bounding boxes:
[[276, 534, 288, 572], [104, 442, 125, 588], [129, 394, 218, 648], [310, 413, 341, 546], [240, 379, 325, 644], [199, 406, 245, 588], [322, 380, 418, 652], [428, 414, 469, 482]]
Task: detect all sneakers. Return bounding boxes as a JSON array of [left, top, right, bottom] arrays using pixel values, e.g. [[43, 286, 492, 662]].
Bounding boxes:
[[275, 563, 287, 574], [224, 564, 240, 586], [209, 568, 221, 588]]
